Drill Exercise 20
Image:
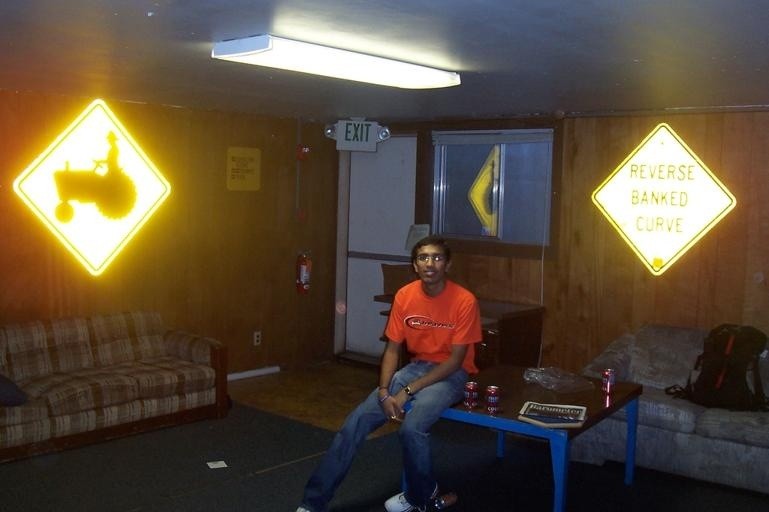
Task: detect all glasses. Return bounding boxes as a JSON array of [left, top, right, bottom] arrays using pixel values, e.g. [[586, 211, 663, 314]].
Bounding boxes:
[[416, 255, 444, 261]]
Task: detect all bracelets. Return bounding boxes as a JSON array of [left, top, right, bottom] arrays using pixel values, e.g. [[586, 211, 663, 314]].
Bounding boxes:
[[378, 386, 388, 390], [377, 394, 392, 406]]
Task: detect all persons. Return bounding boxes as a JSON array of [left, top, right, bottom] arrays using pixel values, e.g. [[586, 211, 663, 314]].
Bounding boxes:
[[296, 236, 483, 512]]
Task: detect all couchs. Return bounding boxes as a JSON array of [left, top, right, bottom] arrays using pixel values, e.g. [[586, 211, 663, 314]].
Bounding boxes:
[[0, 307, 233, 467], [568, 322, 768, 498]]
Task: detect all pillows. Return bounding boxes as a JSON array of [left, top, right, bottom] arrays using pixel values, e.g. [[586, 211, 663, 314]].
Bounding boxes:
[[0, 372, 30, 406]]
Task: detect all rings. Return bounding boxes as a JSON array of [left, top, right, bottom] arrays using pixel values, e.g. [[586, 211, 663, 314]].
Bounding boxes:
[[390, 416, 396, 421]]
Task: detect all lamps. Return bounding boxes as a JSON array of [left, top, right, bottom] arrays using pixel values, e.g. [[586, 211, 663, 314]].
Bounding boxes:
[[209, 33, 463, 92]]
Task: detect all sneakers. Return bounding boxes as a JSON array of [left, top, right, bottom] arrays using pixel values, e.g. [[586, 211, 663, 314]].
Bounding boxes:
[[383, 479, 438, 511]]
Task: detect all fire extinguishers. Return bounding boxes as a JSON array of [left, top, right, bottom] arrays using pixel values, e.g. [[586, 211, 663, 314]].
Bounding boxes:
[[296, 248, 312, 295]]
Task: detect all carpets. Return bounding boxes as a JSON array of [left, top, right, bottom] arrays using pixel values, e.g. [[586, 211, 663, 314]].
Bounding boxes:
[[225, 359, 405, 443]]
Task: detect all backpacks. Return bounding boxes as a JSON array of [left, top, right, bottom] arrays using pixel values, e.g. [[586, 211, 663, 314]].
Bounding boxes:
[[666, 323, 768, 411]]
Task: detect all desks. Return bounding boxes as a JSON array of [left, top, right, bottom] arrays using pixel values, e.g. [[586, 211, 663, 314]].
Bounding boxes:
[[373, 288, 548, 376], [394, 362, 644, 511]]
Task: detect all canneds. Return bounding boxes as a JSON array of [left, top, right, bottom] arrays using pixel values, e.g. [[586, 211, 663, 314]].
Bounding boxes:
[[601, 368, 617, 393], [600, 393, 616, 409], [484, 385, 503, 416], [463, 381, 480, 410]]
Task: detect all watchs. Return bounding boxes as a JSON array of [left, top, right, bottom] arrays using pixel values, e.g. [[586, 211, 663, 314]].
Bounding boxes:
[[403, 384, 415, 396]]
